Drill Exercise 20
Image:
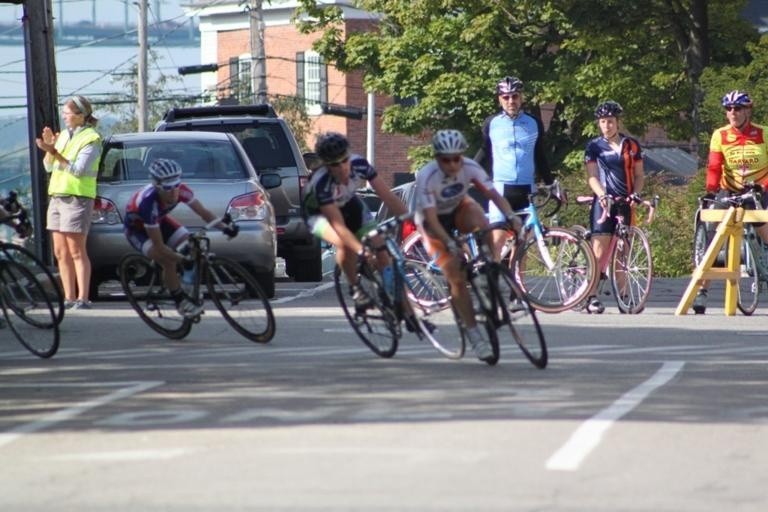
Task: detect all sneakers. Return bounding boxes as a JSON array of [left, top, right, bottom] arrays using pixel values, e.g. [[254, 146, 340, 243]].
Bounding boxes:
[[465, 322, 493, 360], [176, 299, 202, 321], [348, 283, 373, 307], [616, 295, 644, 314], [586, 294, 605, 315], [692, 289, 708, 316], [405, 313, 437, 335], [509, 298, 530, 312], [57, 298, 94, 312]]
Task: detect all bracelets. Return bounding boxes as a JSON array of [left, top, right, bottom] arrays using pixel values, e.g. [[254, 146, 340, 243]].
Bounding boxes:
[[506, 213, 513, 222]]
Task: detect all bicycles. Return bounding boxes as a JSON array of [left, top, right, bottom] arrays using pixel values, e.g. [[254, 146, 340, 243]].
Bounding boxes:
[[0, 192, 65, 357], [556, 193, 661, 314], [691, 182, 766, 317], [116, 206, 276, 346], [431, 213, 547, 369], [399, 185, 602, 314], [333, 213, 466, 361]]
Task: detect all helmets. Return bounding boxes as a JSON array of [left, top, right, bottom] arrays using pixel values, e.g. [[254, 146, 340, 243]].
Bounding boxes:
[[496, 75, 524, 94], [147, 159, 182, 181], [593, 100, 622, 117], [430, 128, 470, 156], [721, 89, 752, 106], [315, 131, 349, 158]]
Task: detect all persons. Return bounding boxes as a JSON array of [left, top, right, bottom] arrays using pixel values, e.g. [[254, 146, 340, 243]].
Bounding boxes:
[[472, 74, 569, 313], [0, 192, 35, 240], [582, 99, 647, 315], [35, 94, 106, 312], [412, 128, 525, 362], [690, 89, 768, 315], [121, 158, 240, 320], [298, 132, 437, 337]]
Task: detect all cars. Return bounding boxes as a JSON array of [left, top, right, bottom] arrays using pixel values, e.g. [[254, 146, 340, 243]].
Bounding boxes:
[[83, 131, 276, 303]]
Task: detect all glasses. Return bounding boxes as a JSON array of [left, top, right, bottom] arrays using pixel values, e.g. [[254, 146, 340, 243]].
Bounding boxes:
[[156, 180, 184, 191], [499, 94, 524, 101], [725, 105, 751, 112], [324, 154, 350, 167]]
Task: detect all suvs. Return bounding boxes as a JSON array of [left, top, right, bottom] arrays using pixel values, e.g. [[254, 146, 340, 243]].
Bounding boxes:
[[156, 104, 325, 282]]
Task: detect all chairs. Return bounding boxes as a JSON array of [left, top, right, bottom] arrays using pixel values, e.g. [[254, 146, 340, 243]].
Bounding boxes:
[[193, 158, 226, 181], [238, 134, 281, 171], [111, 156, 148, 182]]
